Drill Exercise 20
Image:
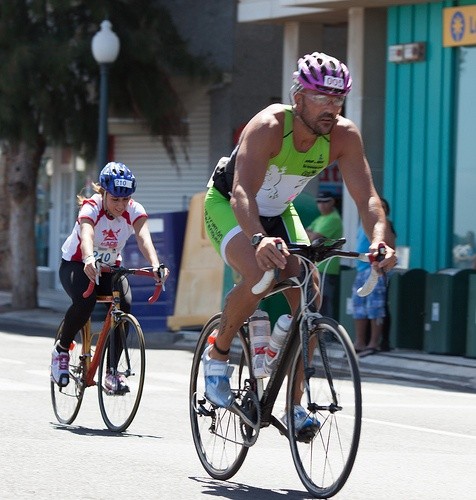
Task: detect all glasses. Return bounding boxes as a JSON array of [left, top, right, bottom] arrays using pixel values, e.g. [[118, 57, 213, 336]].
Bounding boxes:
[[300, 91, 345, 108]]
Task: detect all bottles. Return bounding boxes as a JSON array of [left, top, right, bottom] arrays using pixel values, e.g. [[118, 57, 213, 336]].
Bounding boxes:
[[247, 308, 271, 378], [262, 313, 293, 375]]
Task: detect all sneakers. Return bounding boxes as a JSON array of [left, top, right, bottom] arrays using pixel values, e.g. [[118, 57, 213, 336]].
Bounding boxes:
[[51, 340, 71, 387], [105, 367, 130, 393], [201, 344, 235, 408], [279, 405, 321, 436]]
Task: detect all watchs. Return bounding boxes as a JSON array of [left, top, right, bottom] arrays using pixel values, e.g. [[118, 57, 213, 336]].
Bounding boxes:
[[250, 232, 269, 249]]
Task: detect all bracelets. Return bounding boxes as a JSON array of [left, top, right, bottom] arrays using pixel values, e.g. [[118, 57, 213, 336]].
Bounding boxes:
[[82, 255, 94, 262]]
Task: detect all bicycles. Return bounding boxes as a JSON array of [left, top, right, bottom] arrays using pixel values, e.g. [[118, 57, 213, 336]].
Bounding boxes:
[[189, 234, 395, 498], [51, 262, 163, 436]]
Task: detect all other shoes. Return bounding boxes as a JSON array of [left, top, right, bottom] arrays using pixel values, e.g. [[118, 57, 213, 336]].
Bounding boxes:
[[343, 345, 382, 359]]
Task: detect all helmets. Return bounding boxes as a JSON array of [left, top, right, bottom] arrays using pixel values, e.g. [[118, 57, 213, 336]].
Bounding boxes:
[[98, 161, 138, 197], [292, 52, 353, 96]]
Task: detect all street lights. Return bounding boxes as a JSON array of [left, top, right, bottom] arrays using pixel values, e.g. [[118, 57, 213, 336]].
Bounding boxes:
[[91, 18, 119, 180]]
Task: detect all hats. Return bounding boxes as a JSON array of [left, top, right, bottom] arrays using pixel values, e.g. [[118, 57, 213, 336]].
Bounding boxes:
[[315, 190, 333, 202]]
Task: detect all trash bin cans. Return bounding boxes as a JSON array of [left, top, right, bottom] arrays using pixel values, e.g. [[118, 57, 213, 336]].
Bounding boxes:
[[423, 267, 476, 360], [338, 266, 427, 349]]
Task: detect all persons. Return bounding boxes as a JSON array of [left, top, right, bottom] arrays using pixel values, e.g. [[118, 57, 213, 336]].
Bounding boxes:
[[351, 197, 396, 357], [306, 192, 343, 342], [51, 161, 169, 392], [203, 52, 397, 435]]
